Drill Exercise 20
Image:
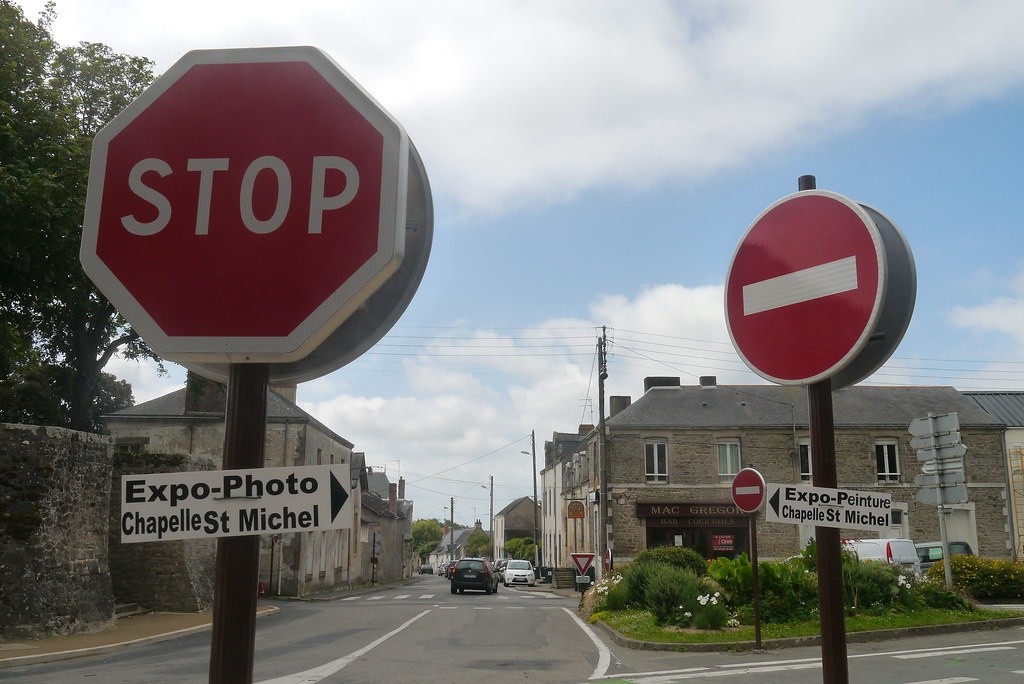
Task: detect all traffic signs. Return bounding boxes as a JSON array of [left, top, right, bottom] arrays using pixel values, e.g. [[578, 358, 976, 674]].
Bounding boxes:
[[120, 463, 355, 546], [765, 482, 895, 534]]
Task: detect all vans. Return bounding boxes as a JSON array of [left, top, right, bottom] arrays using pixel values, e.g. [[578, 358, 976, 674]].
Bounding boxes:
[[840, 538, 923, 576], [914, 540, 974, 579]]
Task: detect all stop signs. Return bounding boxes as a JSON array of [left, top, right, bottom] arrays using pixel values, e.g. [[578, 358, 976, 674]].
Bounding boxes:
[[731, 468, 768, 513], [723, 188, 889, 387], [605, 549, 611, 572], [78, 45, 411, 367]]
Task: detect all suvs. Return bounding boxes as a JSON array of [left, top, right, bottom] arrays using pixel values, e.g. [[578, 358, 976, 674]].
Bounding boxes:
[[449, 556, 499, 595]]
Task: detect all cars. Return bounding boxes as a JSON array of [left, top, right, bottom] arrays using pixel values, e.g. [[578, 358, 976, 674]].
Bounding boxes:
[[503, 560, 536, 587], [419, 565, 432, 575], [774, 554, 812, 575], [493, 559, 508, 583], [437, 560, 459, 580]]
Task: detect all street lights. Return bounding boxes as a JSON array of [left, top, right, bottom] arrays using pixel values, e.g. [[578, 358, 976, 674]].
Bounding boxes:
[[521, 450, 542, 568], [482, 484, 493, 565], [443, 506, 454, 561]]
